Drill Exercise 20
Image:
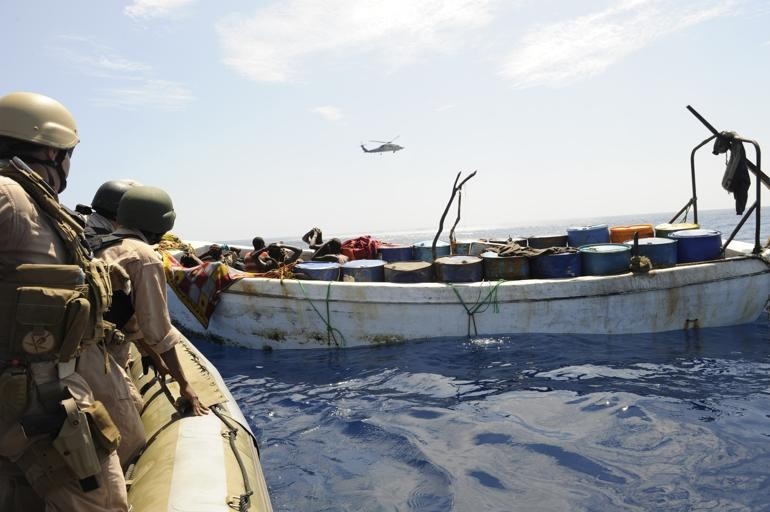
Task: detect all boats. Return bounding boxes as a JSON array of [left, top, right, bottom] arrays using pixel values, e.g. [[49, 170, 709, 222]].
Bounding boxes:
[[166, 236, 770, 352], [0, 320, 275, 510]]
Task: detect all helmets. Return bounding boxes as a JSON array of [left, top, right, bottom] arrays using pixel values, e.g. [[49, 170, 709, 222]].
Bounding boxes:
[[91, 179, 133, 218], [117, 186, 176, 232], [0, 91, 81, 149]]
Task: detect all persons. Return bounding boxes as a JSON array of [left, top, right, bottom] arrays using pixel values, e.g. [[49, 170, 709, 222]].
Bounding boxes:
[[77, 186, 210, 468], [179, 224, 359, 282], [0, 89, 151, 511], [78, 177, 143, 248]]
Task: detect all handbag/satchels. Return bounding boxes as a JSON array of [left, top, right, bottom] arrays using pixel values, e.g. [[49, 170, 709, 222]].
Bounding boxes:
[[1, 262, 103, 364]]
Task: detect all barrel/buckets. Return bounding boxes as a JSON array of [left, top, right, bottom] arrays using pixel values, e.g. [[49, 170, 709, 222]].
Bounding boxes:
[[294, 222, 723, 283]]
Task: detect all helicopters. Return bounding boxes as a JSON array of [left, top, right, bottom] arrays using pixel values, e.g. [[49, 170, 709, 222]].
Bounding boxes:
[[360, 134, 405, 154]]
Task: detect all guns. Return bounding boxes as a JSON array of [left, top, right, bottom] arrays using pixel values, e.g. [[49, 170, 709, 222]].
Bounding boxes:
[[21, 413, 100, 494]]
